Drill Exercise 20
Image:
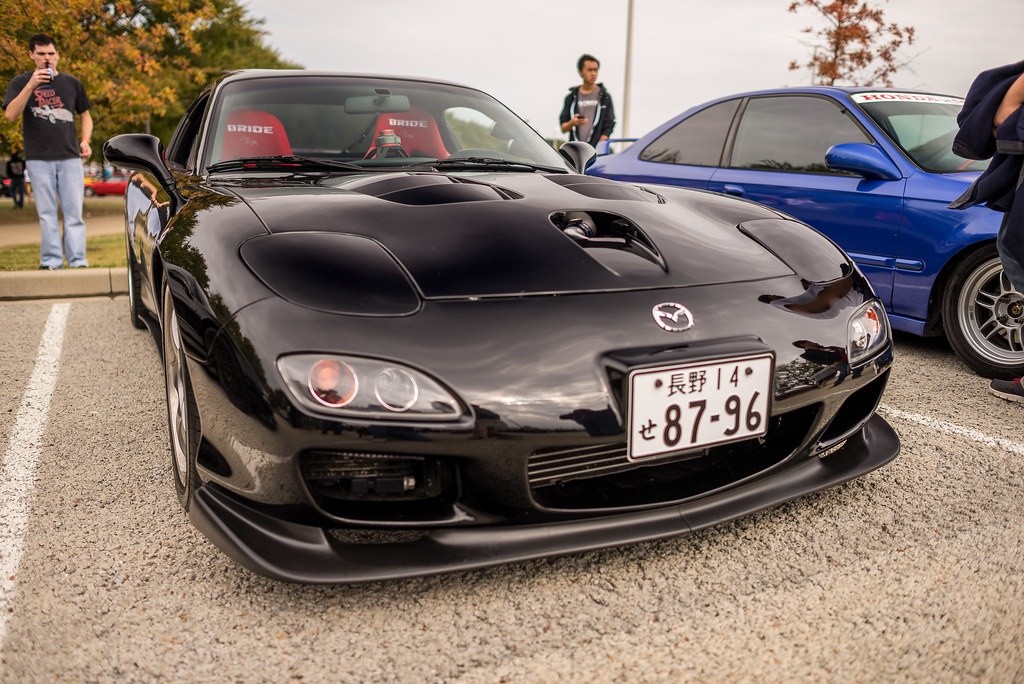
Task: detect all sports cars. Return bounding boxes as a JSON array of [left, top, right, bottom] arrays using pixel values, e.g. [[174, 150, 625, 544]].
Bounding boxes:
[[102, 68, 903, 586]]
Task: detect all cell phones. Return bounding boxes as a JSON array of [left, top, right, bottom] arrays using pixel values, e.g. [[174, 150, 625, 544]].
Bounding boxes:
[[577, 115, 585, 119]]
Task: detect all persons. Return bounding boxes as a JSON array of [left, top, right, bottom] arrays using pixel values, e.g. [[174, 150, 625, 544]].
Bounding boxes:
[[87, 166, 132, 196], [946, 59, 1024, 402], [5, 153, 31, 208], [560, 54, 616, 156], [4, 34, 94, 270]]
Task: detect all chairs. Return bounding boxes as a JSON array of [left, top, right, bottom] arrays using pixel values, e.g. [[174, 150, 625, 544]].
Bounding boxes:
[[364, 103, 451, 160], [219, 107, 301, 166]]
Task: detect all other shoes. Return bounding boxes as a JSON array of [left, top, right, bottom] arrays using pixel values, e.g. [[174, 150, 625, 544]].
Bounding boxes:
[[38, 263, 63, 270]]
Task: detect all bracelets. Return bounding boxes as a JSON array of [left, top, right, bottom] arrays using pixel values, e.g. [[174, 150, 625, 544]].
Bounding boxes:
[[81, 139, 91, 147]]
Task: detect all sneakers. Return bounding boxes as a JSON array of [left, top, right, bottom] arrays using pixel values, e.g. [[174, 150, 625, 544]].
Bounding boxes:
[[989, 378, 1024, 403]]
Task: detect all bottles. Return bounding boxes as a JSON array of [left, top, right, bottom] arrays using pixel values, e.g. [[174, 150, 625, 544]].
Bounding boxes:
[[44, 62, 53, 81]]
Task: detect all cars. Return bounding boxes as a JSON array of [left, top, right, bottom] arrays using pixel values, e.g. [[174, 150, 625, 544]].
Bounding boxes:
[[0, 162, 11, 196], [586, 85, 1024, 380], [85, 173, 130, 197]]
